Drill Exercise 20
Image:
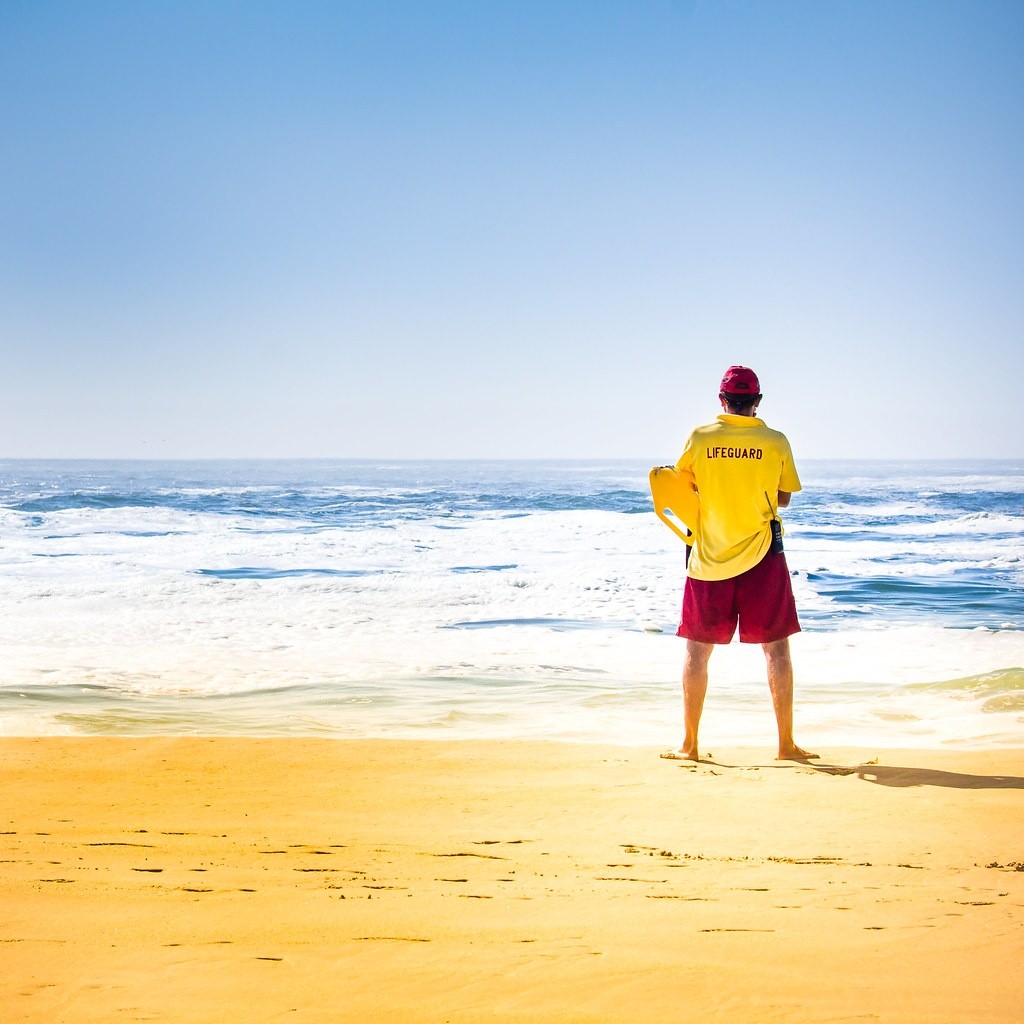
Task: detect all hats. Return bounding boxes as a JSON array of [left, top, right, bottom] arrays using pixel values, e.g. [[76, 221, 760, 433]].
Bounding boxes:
[[720, 366, 760, 402]]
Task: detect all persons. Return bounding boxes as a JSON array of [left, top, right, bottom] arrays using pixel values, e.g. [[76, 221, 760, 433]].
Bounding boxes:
[[660, 365, 820, 761]]
